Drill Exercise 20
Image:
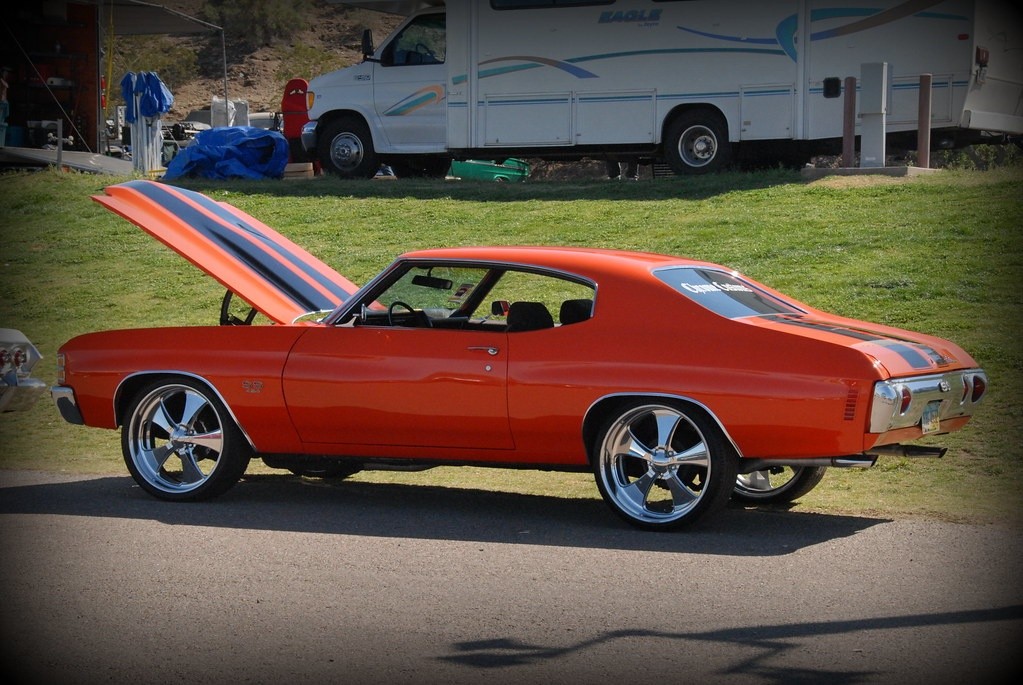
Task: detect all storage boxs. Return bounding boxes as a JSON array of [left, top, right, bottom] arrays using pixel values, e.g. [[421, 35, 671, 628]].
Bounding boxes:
[[285, 163, 313, 179]]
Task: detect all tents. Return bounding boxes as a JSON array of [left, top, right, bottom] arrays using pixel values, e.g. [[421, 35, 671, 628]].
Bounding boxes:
[[119, 71, 174, 176]]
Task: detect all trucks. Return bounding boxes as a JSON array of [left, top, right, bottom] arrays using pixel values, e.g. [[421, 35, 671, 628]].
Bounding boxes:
[[301, 1, 1023, 183]]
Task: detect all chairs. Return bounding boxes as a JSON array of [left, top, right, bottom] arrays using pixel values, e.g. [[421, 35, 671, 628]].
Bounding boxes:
[[559, 299, 593, 325], [173, 123, 191, 151], [507, 302, 553, 331]]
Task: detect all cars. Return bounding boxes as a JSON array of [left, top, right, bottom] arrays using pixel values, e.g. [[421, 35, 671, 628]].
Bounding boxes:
[[48, 179, 990, 533]]
